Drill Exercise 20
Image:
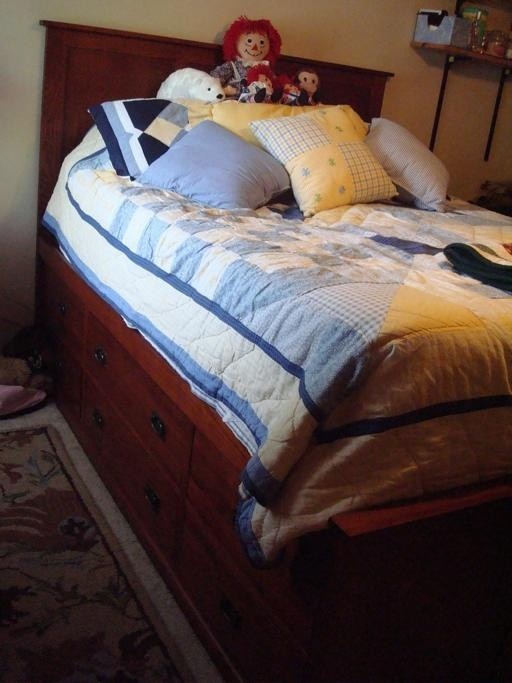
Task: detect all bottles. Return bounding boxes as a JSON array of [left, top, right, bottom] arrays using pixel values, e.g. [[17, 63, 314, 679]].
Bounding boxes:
[[486, 29, 506, 57]]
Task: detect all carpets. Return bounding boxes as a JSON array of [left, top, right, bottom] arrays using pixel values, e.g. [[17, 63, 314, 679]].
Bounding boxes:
[[0, 352, 224, 680]]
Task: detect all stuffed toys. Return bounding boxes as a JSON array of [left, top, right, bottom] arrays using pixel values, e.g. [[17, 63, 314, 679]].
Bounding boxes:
[[154, 17, 323, 109]]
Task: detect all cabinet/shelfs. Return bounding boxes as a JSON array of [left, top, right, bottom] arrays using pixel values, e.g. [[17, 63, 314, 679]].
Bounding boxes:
[[28, 18, 512, 683]]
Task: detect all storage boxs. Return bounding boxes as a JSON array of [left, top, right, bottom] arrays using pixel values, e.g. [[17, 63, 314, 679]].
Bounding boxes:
[[411, 12, 472, 49]]
